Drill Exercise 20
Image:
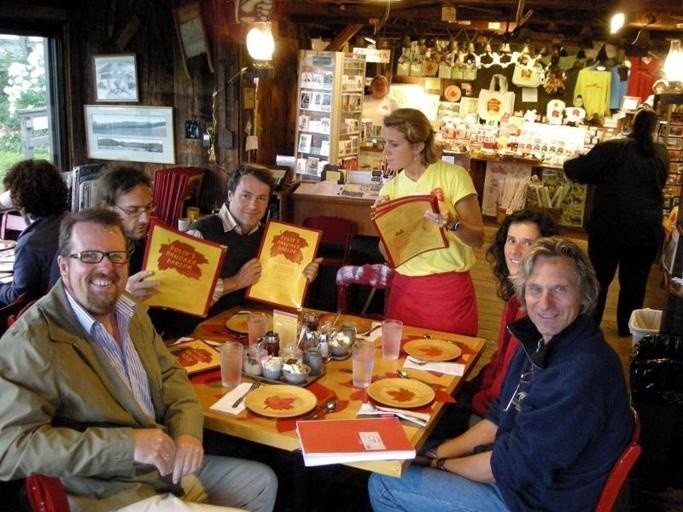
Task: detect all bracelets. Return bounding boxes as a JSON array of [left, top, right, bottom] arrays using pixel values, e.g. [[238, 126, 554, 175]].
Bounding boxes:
[[435, 457, 447, 474], [425, 448, 436, 459]]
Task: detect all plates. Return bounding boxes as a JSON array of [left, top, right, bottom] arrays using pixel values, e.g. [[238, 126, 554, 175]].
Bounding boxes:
[[224, 311, 274, 335], [317, 312, 372, 333], [245, 383, 318, 418], [366, 378, 435, 408], [403, 337, 462, 364]]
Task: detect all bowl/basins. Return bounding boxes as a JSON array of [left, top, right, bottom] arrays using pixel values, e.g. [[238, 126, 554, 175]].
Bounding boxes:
[[260, 369, 282, 379], [282, 364, 311, 383], [329, 343, 350, 357]]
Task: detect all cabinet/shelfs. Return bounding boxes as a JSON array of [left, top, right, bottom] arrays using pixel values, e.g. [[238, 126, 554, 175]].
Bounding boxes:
[[654, 94, 682, 217]]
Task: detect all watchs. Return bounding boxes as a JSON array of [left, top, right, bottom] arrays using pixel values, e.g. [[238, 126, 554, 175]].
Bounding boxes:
[[445, 214, 463, 232]]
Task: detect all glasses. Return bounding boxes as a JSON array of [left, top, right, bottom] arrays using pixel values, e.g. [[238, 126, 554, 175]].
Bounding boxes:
[[115, 198, 158, 218], [68, 251, 132, 265]]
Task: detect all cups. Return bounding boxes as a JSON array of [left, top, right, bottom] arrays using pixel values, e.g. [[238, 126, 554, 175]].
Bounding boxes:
[[247, 311, 267, 347], [351, 342, 375, 389], [218, 342, 243, 389], [381, 319, 403, 360]]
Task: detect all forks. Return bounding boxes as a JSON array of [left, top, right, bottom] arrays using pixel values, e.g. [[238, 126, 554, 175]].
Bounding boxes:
[[369, 400, 429, 424], [232, 378, 259, 409], [361, 322, 382, 337]]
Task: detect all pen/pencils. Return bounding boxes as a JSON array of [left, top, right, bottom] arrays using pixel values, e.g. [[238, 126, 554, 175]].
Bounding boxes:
[[381, 165, 394, 178], [337, 188, 342, 195]]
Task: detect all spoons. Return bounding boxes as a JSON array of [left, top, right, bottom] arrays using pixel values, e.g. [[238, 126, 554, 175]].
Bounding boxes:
[[396, 369, 449, 389], [306, 397, 336, 420]]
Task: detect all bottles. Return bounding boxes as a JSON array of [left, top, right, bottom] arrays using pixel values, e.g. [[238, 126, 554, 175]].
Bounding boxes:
[[302, 311, 317, 333], [306, 348, 323, 375]]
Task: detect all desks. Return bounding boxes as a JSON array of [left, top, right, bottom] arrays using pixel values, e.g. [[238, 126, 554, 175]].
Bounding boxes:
[[292, 182, 382, 237]]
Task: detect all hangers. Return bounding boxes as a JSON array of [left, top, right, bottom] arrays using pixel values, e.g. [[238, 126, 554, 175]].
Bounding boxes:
[[590, 51, 659, 79]]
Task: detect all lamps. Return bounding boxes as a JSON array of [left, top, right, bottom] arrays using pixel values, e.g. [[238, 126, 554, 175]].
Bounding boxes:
[[245, 25, 275, 80]]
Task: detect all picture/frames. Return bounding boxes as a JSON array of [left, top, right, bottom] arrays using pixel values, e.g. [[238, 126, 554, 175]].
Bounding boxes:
[[91, 53, 139, 103], [83, 104, 175, 165]]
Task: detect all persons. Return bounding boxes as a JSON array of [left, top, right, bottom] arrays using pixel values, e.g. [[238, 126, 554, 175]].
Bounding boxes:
[[372, 107, 486, 338], [369, 235, 636, 512], [90, 161, 191, 341], [428, 208, 557, 436], [1, 208, 279, 512], [0, 157, 69, 323], [563, 108, 671, 338], [182, 161, 324, 338], [362, 74, 398, 124]]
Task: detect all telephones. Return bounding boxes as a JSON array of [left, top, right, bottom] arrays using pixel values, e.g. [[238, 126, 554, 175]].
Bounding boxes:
[[272, 166, 290, 190]]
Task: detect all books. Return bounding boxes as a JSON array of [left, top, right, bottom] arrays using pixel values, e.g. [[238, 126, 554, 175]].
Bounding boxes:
[[296, 418, 417, 469], [59, 163, 107, 213], [152, 165, 205, 226]]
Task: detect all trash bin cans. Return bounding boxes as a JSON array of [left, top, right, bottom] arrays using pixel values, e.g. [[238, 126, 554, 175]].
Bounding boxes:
[[632, 332, 683, 455], [629, 308, 663, 349]]
